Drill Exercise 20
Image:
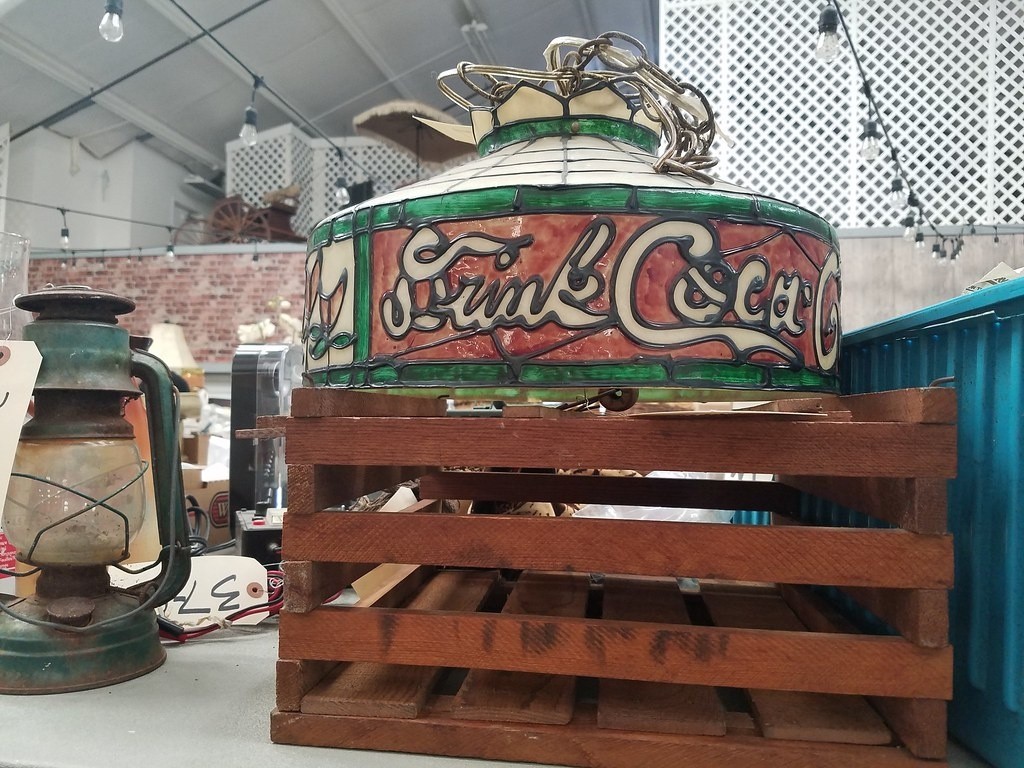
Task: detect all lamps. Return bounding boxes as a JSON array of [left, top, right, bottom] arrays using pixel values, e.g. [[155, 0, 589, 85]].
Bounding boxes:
[[0, 286, 198, 695], [150, 320, 197, 374]]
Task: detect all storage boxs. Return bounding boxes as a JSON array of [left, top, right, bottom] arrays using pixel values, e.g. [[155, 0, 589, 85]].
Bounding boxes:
[[0, 369, 232, 602], [270, 377, 959, 768], [731, 279, 1024, 768]]
